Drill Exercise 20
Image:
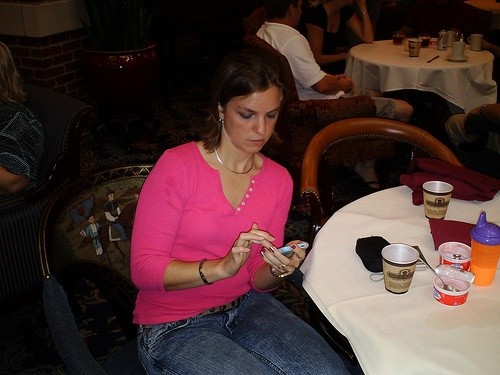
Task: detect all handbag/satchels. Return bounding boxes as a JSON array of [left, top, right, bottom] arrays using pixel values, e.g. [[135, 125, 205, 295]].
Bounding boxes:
[[356, 235, 391, 273]]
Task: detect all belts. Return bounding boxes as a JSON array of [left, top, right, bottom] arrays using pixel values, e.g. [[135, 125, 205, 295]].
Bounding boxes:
[[196, 300, 236, 319]]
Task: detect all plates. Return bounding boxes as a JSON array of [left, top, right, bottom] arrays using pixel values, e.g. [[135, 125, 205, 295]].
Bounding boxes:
[[447, 54, 469, 61]]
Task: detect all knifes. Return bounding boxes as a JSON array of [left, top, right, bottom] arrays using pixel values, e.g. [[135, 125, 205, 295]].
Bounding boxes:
[[427, 55, 439, 63]]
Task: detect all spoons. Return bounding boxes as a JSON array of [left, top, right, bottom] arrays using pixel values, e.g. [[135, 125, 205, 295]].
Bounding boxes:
[[412, 245, 460, 292]]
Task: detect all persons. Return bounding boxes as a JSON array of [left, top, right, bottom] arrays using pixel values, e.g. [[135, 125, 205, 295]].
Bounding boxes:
[[130, 52, 352, 375], [392, 0, 464, 36], [444, 37, 500, 166], [0, 40, 44, 194], [303, 0, 373, 75], [255, 0, 414, 189]]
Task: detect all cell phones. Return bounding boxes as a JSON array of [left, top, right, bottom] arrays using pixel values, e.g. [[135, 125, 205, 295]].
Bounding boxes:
[[274, 242, 310, 259]]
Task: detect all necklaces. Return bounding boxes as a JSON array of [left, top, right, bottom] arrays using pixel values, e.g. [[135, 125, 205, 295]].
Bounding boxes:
[[215, 149, 255, 174]]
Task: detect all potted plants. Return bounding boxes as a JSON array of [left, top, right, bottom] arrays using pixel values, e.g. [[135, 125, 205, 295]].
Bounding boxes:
[[79, 0, 164, 118]]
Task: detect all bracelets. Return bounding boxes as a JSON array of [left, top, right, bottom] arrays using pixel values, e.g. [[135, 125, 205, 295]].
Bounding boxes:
[[270, 265, 295, 277], [480, 104, 487, 115], [360, 11, 368, 15], [199, 258, 214, 285]]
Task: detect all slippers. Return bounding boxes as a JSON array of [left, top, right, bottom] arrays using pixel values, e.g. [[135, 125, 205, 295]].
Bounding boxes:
[[359, 175, 379, 194]]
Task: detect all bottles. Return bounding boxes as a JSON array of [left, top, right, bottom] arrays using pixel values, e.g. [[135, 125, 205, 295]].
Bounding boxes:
[[469, 212, 500, 286]]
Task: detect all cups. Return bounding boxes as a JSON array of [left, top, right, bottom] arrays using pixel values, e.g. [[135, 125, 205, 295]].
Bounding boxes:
[[468, 34, 483, 51], [392, 30, 465, 57], [423, 180, 454, 220], [438, 242, 472, 272], [382, 244, 419, 295], [431, 274, 470, 306]]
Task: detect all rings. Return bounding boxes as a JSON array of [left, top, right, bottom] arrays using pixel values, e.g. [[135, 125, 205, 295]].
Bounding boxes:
[[278, 265, 285, 270]]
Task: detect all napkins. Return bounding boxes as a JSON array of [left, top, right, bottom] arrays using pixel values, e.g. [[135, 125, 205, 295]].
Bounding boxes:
[[400, 157, 500, 206]]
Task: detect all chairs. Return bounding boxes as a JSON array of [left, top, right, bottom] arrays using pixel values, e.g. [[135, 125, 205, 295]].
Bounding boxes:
[[0, 7, 460, 375]]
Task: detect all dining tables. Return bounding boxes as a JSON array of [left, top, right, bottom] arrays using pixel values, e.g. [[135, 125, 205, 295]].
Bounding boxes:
[[344, 39, 497, 114], [301, 185, 500, 375]]
